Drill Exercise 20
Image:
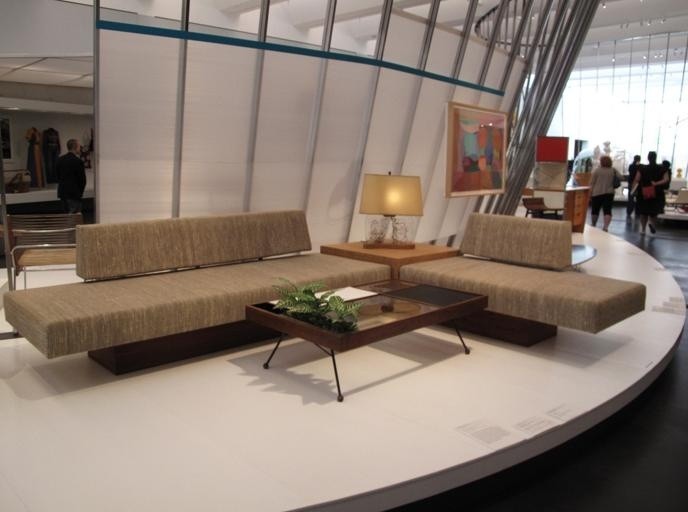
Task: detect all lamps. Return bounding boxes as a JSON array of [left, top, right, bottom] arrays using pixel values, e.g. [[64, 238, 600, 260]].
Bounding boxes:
[[359, 171, 423, 248]]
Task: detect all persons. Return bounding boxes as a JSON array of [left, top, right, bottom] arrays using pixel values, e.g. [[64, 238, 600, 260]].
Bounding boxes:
[[660, 158, 672, 191], [628, 151, 670, 233], [625, 155, 643, 227], [585, 154, 621, 233], [54, 136, 87, 215]]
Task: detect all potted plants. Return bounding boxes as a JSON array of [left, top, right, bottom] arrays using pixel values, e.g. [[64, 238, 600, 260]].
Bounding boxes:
[[573, 157, 592, 185]]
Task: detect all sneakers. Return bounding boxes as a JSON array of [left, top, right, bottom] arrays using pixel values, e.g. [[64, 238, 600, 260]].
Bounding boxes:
[[648, 223, 656, 233]]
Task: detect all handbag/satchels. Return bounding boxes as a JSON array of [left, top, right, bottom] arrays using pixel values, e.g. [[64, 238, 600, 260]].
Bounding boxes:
[[642, 186, 655, 198], [613, 174, 622, 189]]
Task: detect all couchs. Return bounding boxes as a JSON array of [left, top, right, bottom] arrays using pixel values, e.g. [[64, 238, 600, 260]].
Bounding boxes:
[[399, 213, 645, 347], [4, 211, 392, 375]]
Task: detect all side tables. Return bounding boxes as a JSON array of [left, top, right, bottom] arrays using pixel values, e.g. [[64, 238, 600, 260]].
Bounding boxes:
[[320, 241, 461, 279]]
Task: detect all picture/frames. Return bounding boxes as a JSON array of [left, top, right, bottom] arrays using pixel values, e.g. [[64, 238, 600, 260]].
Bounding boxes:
[[445, 101, 507, 197]]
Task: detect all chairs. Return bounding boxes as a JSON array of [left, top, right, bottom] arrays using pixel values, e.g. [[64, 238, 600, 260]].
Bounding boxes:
[[669, 187, 688, 209], [521, 197, 564, 218], [7, 212, 83, 338]]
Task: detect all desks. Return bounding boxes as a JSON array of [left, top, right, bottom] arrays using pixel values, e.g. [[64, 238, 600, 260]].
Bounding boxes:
[[534, 187, 592, 232]]
